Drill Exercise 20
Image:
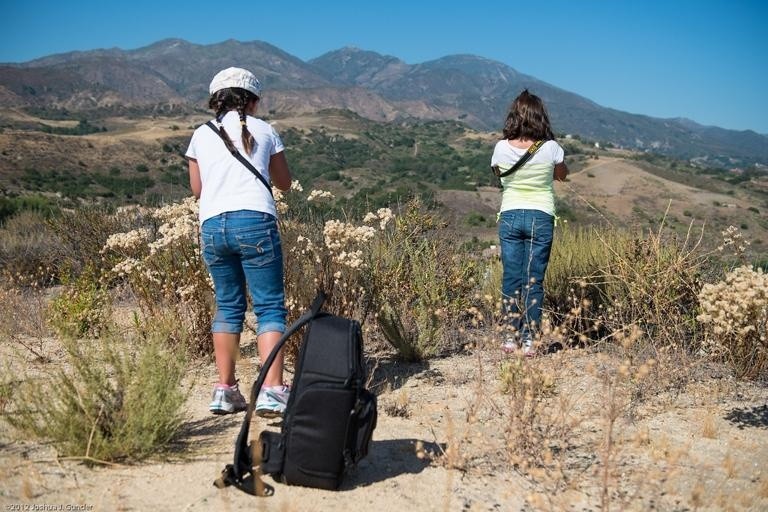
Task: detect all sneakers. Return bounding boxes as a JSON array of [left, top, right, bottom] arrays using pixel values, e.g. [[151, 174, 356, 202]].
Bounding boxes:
[[503, 339, 538, 359], [210, 382, 248, 414], [253, 383, 292, 419]]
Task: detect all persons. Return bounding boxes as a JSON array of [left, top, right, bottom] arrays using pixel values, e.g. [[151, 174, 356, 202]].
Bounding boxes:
[[491, 90, 567, 357], [183, 67, 291, 417]]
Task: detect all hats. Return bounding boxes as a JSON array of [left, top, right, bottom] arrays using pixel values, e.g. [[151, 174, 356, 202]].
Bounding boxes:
[[207, 66, 262, 98]]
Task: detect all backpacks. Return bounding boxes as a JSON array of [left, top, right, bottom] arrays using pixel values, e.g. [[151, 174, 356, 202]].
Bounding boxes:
[[233, 291, 378, 491]]
